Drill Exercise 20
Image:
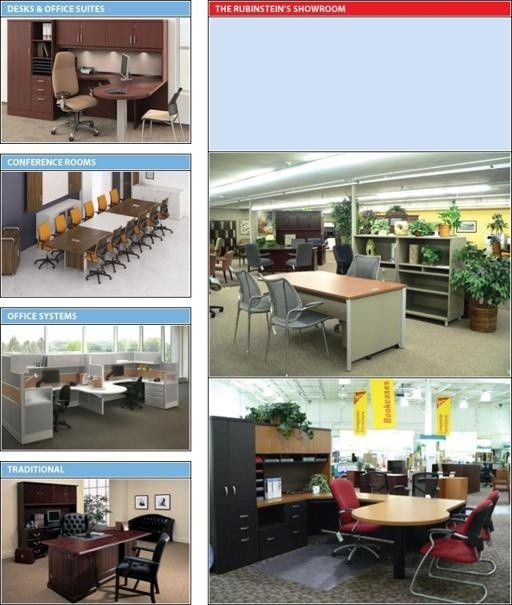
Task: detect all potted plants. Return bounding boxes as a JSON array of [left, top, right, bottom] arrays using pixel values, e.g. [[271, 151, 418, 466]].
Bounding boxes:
[[332, 193, 511, 332], [240, 401, 315, 441], [83, 494, 112, 538]]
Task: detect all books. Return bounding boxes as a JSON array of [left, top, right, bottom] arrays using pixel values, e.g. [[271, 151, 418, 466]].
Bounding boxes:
[[36, 23, 53, 57]]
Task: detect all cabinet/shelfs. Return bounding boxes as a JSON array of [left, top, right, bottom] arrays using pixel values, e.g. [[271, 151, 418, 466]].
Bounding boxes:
[[2, 226, 21, 276], [56, 20, 168, 52], [7, 19, 61, 120], [210, 415, 333, 575], [145, 383, 164, 410], [324, 227, 336, 240], [210, 220, 237, 256], [18, 482, 78, 559], [271, 210, 321, 244]]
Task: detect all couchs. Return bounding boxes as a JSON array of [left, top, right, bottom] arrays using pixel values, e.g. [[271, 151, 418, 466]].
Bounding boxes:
[[128, 514, 174, 544]]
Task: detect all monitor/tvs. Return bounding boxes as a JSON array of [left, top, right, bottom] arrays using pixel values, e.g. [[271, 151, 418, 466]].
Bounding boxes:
[[36, 369, 60, 387], [47, 510, 62, 523], [120, 53, 132, 82], [105, 364, 124, 381]]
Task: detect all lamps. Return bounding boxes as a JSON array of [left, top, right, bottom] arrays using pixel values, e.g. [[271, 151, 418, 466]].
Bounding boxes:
[[337, 377, 492, 409]]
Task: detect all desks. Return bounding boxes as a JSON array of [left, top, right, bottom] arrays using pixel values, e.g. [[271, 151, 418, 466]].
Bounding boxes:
[[260, 270, 408, 373], [79, 73, 163, 142], [133, 184, 186, 219], [42, 529, 152, 603]]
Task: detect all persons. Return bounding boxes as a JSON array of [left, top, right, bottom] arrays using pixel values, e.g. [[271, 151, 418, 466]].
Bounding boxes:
[[323, 232, 336, 250]]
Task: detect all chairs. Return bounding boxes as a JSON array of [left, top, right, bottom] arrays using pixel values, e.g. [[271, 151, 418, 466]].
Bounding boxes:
[[115, 532, 170, 603], [34, 189, 173, 285], [51, 51, 100, 141], [331, 457, 511, 605], [51, 385, 72, 432], [117, 376, 143, 410], [141, 87, 187, 142], [59, 512, 88, 538], [209, 236, 332, 365]]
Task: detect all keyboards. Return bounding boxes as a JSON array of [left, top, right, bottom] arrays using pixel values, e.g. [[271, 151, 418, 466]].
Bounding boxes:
[[108, 88, 129, 94]]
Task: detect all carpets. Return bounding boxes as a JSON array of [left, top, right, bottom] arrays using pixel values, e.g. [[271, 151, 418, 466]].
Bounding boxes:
[[254, 542, 373, 592]]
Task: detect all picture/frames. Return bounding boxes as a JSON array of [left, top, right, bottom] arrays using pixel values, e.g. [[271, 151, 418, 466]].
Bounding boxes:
[[239, 219, 250, 235], [153, 495, 171, 510], [146, 172, 154, 180], [134, 495, 148, 510]]
